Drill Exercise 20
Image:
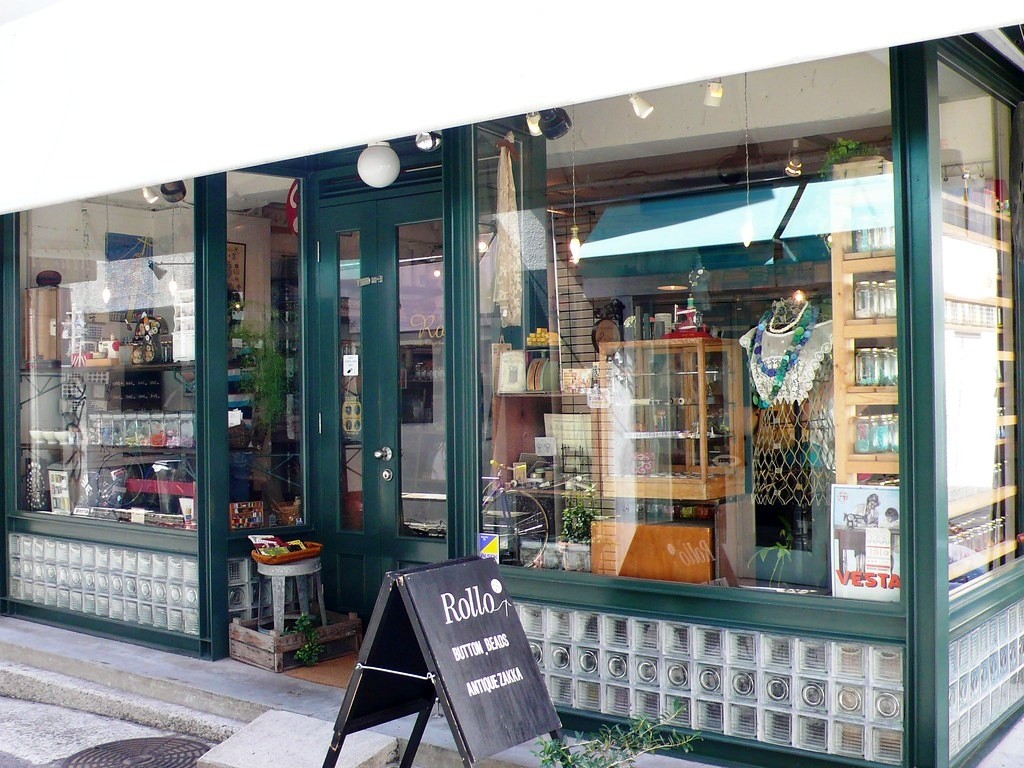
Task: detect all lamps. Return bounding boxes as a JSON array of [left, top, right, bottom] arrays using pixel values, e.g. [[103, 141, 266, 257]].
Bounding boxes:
[[538, 108, 572, 140], [702, 78, 724, 109], [161, 180, 187, 202], [784, 136, 803, 178], [718, 131, 758, 185], [526, 111, 543, 136], [569, 225, 580, 257], [628, 94, 656, 119], [415, 132, 442, 152], [140, 186, 158, 203], [356, 142, 401, 188], [147, 261, 194, 281]]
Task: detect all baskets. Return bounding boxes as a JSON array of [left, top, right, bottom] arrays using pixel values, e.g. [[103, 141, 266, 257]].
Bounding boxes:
[[271, 499, 301, 524]]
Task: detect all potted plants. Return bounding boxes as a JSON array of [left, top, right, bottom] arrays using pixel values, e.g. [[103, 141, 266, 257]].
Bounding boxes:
[[258, 614, 326, 668], [818, 137, 887, 179], [558, 484, 608, 572]]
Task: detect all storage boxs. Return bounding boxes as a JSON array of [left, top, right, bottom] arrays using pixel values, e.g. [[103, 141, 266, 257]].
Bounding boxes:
[[227, 501, 264, 529], [831, 159, 893, 181], [228, 610, 360, 673], [86, 411, 195, 449], [48, 467, 129, 523]]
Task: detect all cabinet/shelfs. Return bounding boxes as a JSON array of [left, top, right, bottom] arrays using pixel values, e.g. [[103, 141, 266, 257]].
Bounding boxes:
[[18, 360, 196, 455], [589, 338, 755, 585], [830, 183, 1013, 592], [500, 391, 591, 497]]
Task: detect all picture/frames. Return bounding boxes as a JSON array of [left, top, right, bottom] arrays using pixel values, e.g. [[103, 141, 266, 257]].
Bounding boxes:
[[513, 462, 527, 484], [226, 240, 246, 308]]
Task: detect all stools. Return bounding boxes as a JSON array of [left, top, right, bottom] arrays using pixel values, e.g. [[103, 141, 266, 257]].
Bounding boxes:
[[257, 558, 328, 632]]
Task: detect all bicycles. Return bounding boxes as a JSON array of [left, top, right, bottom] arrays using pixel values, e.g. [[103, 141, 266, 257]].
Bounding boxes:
[[482, 460, 550, 568]]
